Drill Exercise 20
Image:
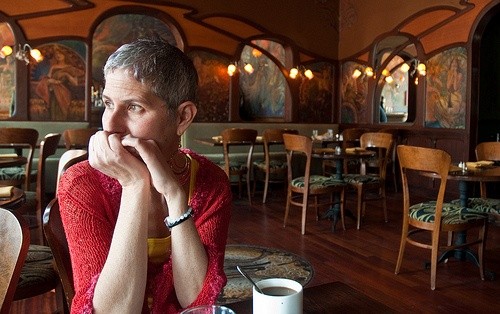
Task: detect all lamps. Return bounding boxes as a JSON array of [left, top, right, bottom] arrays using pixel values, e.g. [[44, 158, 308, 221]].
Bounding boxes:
[[400, 58, 427, 85], [378, 70, 392, 85], [290, 65, 314, 81], [0, 43, 44, 65], [351, 67, 376, 81], [227, 59, 254, 77]]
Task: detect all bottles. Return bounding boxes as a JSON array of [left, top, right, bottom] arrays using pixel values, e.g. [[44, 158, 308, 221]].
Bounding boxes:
[[459, 161, 466, 172]]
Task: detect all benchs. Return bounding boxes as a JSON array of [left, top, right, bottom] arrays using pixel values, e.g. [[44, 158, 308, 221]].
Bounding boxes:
[[181, 123, 341, 183], [0, 121, 90, 192]]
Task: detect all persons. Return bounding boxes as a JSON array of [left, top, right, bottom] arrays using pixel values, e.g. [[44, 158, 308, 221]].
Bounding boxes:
[[57, 38, 233, 314]]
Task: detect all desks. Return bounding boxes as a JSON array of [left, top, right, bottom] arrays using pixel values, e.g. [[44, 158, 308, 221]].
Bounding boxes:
[[313, 139, 360, 154], [303, 151, 377, 233], [0, 155, 27, 167], [420, 167, 500, 281], [0, 185, 26, 210], [220, 281, 400, 314], [194, 136, 284, 153]]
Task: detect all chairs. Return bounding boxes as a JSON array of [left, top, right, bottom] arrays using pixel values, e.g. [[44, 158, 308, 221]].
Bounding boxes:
[[0, 128, 99, 314], [220, 128, 500, 291]]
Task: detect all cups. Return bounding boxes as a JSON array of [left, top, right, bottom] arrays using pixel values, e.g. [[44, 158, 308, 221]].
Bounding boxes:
[[328, 129, 333, 139], [313, 129, 318, 140], [253, 278, 304, 314], [179, 305, 235, 314]]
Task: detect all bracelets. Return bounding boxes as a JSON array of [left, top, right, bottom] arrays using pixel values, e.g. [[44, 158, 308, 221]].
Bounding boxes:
[[163, 205, 194, 228]]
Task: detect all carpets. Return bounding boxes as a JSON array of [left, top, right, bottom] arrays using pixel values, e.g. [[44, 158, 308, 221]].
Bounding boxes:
[[221, 244, 314, 305]]
